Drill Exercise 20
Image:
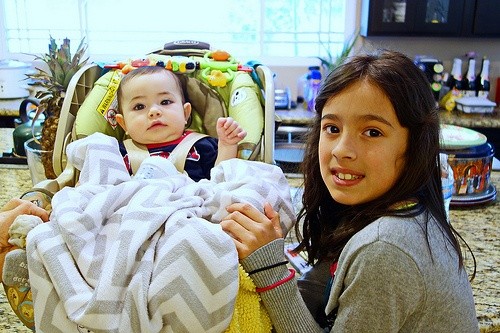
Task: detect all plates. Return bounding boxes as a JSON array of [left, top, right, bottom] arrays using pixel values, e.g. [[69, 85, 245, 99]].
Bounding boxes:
[[450, 184, 496, 206]]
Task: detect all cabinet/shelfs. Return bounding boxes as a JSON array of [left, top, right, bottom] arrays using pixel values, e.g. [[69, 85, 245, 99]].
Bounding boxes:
[[359, 0, 500, 38]]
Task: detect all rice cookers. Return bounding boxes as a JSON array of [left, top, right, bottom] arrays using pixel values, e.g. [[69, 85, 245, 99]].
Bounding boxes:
[[0, 57, 39, 99]]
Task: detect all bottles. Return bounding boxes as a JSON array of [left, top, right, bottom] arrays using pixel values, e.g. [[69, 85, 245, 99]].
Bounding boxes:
[[447, 58, 462, 91], [309, 71, 322, 113], [462, 58, 476, 96], [477, 59, 490, 99]]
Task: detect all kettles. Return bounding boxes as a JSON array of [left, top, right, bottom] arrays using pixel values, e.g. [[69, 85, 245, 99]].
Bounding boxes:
[[13, 98, 47, 159]]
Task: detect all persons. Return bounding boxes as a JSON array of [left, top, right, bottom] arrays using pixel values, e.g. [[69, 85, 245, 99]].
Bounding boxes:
[[219, 48, 480, 332], [115, 66, 247, 183], [0, 198, 50, 283]]
